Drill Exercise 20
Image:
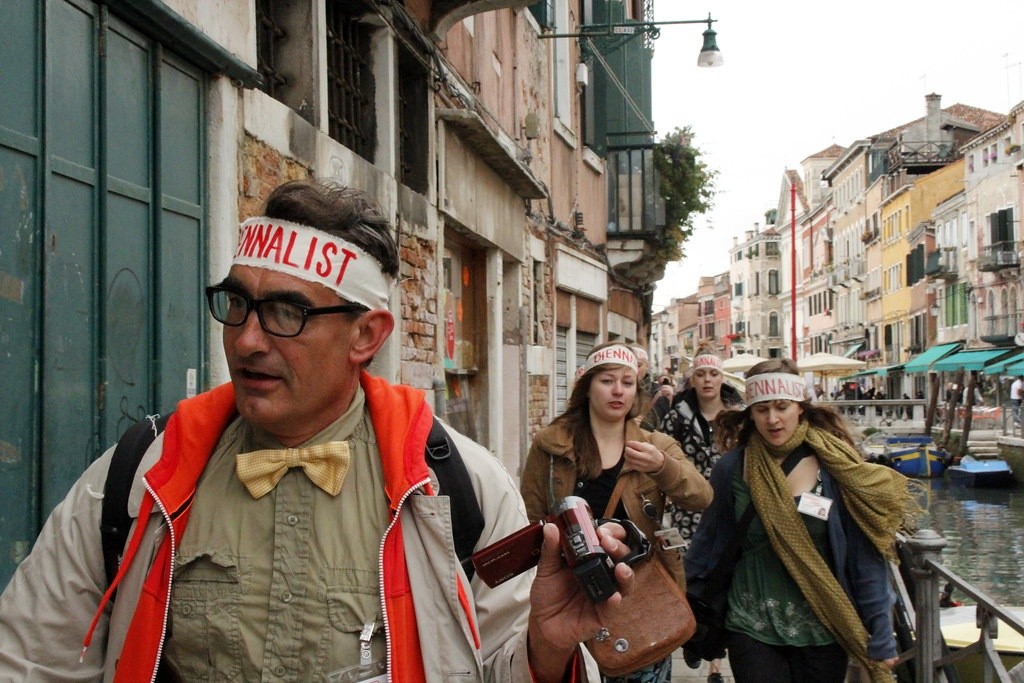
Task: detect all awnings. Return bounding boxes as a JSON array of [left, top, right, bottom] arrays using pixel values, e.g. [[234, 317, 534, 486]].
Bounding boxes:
[[839, 363, 906, 380], [985, 352, 1024, 375], [935, 350, 1012, 370], [845, 344, 862, 358], [905, 343, 962, 372]]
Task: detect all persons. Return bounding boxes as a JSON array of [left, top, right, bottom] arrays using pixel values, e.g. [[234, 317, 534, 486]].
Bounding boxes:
[[815, 384, 885, 414], [943, 379, 996, 407], [632, 347, 745, 683], [0, 180, 634, 683], [902, 391, 923, 415], [1011, 376, 1024, 427], [683, 358, 928, 683], [521, 341, 714, 683]]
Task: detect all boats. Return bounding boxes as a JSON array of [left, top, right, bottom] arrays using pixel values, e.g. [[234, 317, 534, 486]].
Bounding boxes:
[[725, 335, 1024, 484], [861, 431, 946, 478], [948, 455, 1013, 489]]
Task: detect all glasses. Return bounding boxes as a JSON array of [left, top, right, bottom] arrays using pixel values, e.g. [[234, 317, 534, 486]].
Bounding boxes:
[[204, 280, 368, 337]]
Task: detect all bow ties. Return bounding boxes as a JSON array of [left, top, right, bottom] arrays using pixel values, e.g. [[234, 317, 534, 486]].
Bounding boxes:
[[234, 440, 351, 501]]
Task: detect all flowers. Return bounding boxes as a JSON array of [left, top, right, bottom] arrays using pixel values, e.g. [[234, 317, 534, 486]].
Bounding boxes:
[[969, 146, 1011, 169]]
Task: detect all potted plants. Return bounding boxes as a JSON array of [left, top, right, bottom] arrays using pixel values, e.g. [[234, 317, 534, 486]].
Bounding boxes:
[[1011, 145, 1022, 152], [860, 231, 872, 243]]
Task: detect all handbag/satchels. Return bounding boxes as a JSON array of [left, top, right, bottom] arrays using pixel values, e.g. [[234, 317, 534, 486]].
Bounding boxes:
[[692, 564, 738, 661], [582, 557, 696, 676]]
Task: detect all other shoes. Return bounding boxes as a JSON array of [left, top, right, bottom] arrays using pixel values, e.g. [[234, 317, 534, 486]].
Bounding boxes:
[[707, 671, 724, 683], [683, 643, 701, 669]]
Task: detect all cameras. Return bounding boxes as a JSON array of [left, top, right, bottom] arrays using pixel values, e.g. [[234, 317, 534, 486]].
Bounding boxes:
[[472, 495, 618, 605]]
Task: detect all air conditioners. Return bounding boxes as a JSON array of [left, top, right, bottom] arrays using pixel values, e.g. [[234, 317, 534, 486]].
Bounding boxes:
[[997, 251, 1017, 266]]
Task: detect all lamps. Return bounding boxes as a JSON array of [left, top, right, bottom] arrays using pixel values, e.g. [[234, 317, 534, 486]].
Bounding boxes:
[[577, 11, 726, 68]]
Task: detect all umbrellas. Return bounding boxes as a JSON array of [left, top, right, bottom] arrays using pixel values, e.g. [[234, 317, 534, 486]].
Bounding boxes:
[[723, 353, 767, 371], [798, 353, 866, 398]]
